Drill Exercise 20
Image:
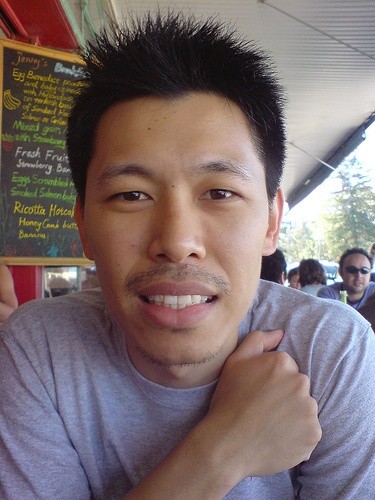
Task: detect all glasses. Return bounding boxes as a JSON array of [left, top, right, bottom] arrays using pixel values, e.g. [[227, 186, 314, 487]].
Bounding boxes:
[[342, 266, 371, 274]]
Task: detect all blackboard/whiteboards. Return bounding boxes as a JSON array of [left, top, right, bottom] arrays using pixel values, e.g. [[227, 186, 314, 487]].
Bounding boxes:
[[0, 39, 134, 266]]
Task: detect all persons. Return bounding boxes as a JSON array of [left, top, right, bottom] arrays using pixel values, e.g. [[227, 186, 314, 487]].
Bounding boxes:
[[1, 0, 375, 500], [260, 247, 288, 287], [287, 266, 305, 290], [317, 247, 374, 310], [297, 257, 329, 297], [0, 256, 20, 323]]
